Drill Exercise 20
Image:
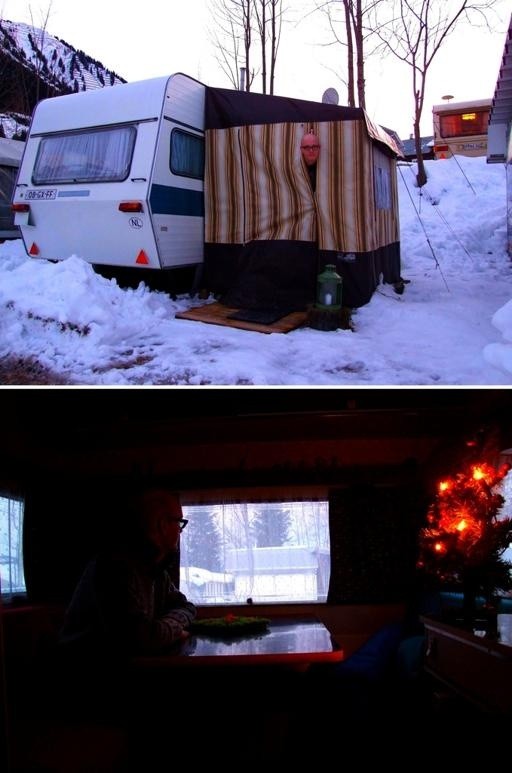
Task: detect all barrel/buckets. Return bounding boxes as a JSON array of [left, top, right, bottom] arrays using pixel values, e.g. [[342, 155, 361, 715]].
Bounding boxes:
[[314, 266, 344, 312]]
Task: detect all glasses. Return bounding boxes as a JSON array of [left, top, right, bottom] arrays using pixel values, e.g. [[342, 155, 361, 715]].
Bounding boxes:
[[174, 517, 187, 528], [301, 144, 320, 151]]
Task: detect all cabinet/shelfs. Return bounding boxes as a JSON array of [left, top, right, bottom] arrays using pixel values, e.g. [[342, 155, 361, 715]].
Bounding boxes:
[[412, 618, 510, 772]]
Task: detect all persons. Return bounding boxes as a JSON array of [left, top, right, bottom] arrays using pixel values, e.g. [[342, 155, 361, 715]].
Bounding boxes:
[[300, 134, 321, 193], [51, 490, 198, 725]]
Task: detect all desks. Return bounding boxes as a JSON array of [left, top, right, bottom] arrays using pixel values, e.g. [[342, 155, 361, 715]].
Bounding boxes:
[[137, 612, 352, 669]]
